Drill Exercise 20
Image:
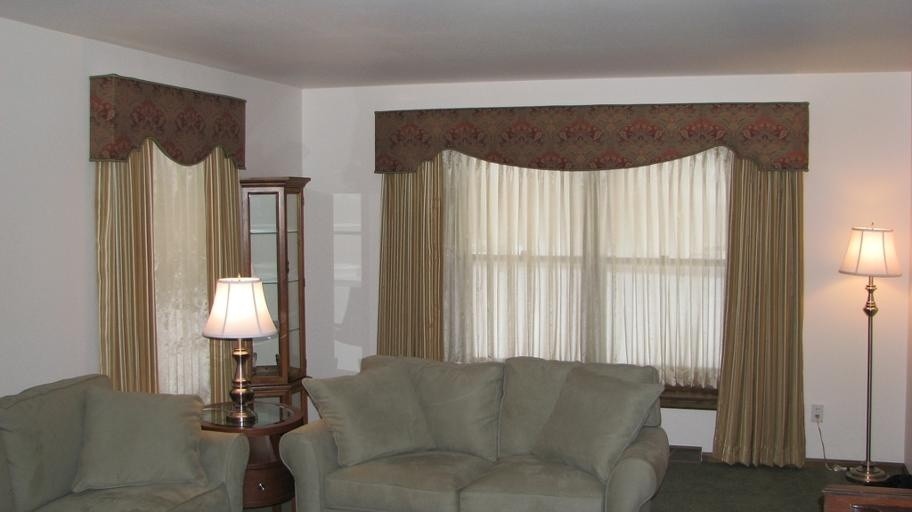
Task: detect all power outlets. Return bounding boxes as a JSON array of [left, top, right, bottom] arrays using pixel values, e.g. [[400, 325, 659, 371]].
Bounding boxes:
[[811, 404, 824, 423]]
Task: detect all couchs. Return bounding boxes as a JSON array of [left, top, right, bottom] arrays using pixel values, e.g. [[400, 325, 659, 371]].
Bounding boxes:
[[278, 355, 671, 511], [0, 374, 251, 512]]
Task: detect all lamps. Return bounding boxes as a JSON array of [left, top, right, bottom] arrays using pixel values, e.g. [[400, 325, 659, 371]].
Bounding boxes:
[[202, 274, 278, 423], [838, 226, 903, 483]]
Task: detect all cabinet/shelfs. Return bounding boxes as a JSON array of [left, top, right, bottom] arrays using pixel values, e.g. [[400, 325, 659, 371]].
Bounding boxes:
[[239, 176, 312, 424]]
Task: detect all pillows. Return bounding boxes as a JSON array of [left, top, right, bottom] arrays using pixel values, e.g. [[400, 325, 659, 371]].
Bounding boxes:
[[529, 366, 665, 482], [72, 386, 208, 495], [301, 366, 437, 467]]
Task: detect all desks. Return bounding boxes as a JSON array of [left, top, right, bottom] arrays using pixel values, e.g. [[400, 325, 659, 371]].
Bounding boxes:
[[201, 400, 304, 509]]
